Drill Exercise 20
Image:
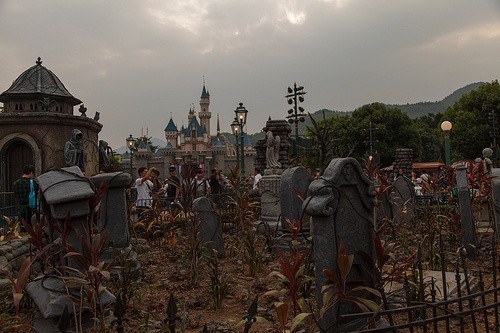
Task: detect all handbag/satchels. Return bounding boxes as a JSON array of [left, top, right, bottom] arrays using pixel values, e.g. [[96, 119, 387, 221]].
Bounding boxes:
[[29, 179, 39, 209]]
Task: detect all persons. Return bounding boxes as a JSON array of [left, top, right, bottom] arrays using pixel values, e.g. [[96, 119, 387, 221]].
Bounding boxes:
[[135, 163, 263, 223], [378, 167, 435, 195], [12, 164, 40, 234]]
